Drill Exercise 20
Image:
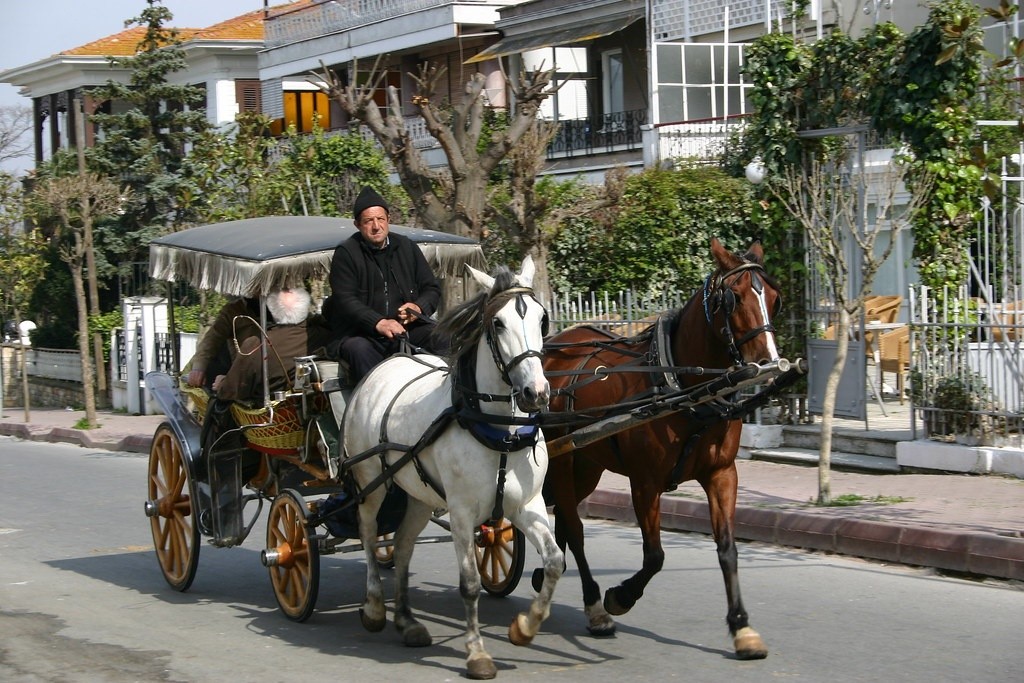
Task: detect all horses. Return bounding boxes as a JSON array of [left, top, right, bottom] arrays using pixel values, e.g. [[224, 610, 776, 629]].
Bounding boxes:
[[344, 254, 567, 678], [531, 234, 790, 657]]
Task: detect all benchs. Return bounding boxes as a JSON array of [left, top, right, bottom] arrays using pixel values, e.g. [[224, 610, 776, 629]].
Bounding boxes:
[[231, 387, 306, 452], [309, 360, 352, 380], [177, 374, 213, 415]]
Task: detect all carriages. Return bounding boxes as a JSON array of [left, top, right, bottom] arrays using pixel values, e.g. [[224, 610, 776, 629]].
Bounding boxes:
[[144, 216, 810, 680]]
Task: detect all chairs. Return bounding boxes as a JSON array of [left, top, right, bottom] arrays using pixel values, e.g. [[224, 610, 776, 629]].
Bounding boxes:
[[966, 296, 1024, 341], [824, 295, 909, 405]]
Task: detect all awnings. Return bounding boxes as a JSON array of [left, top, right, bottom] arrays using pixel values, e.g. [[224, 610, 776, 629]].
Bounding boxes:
[[462, 13, 648, 108]]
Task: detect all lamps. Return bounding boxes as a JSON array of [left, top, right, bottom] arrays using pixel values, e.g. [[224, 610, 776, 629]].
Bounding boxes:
[[743, 154, 768, 185]]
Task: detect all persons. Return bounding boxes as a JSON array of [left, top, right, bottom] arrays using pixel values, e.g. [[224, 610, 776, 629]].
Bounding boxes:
[[197, 286, 322, 471], [187, 297, 276, 389], [320, 185, 452, 391]]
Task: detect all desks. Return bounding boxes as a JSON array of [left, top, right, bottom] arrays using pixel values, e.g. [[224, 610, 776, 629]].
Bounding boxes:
[[851, 323, 907, 398]]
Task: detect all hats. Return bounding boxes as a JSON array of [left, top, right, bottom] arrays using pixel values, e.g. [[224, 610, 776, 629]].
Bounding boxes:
[[354, 185, 389, 220]]
